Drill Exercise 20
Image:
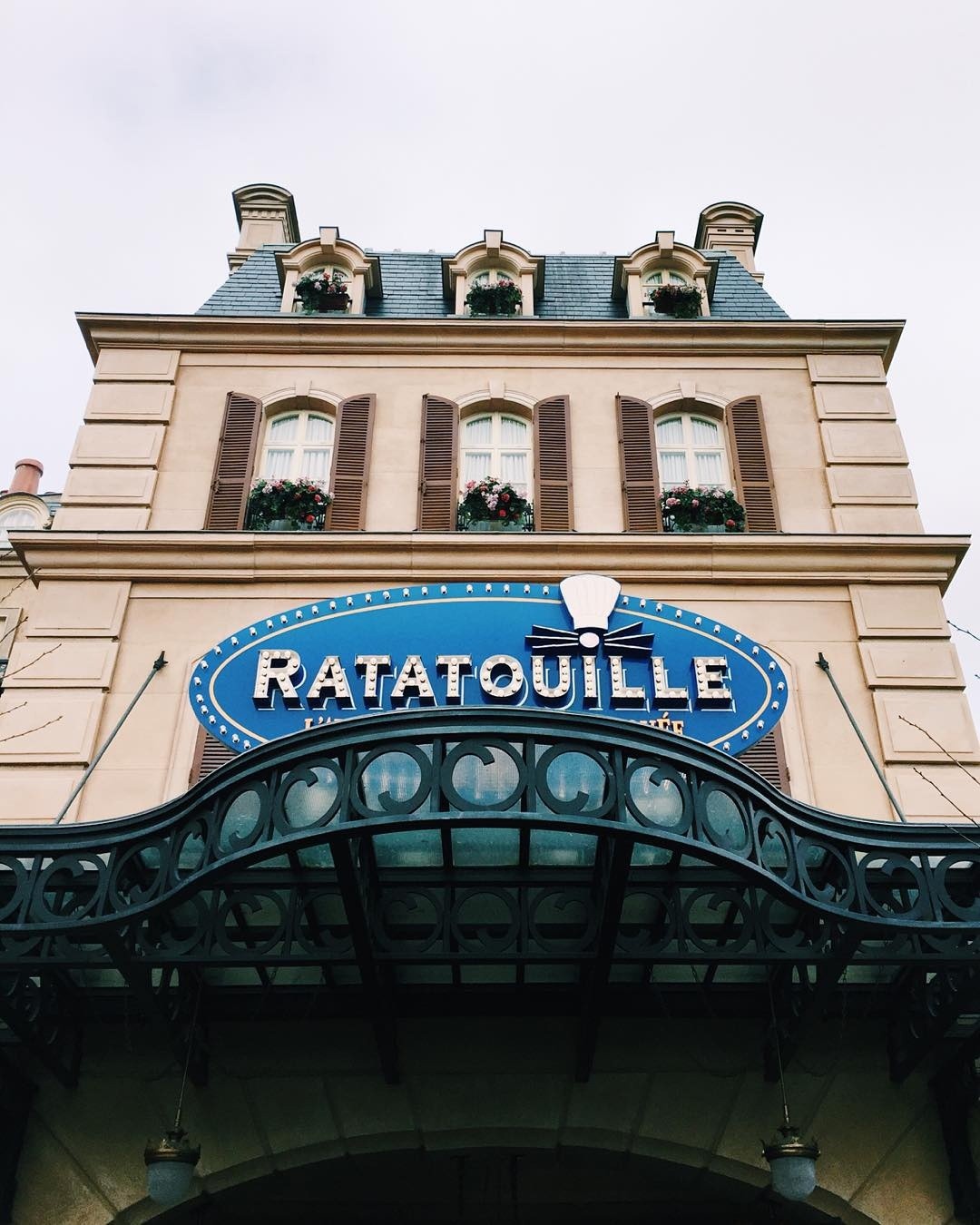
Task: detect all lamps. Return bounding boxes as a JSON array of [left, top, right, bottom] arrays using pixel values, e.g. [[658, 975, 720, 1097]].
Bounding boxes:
[[143, 970, 202, 1203], [760, 967, 820, 1198]]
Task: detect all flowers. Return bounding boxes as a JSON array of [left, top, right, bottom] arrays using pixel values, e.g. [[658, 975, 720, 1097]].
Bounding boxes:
[[659, 487, 745, 532], [250, 480, 334, 532], [650, 284, 702, 321], [296, 272, 351, 314], [463, 279, 522, 319], [457, 481, 527, 525]]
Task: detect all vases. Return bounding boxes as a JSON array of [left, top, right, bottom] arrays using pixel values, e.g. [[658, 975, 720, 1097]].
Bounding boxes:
[[653, 299, 673, 312], [679, 522, 726, 534], [318, 294, 349, 311], [470, 519, 521, 532], [266, 518, 300, 532]]
